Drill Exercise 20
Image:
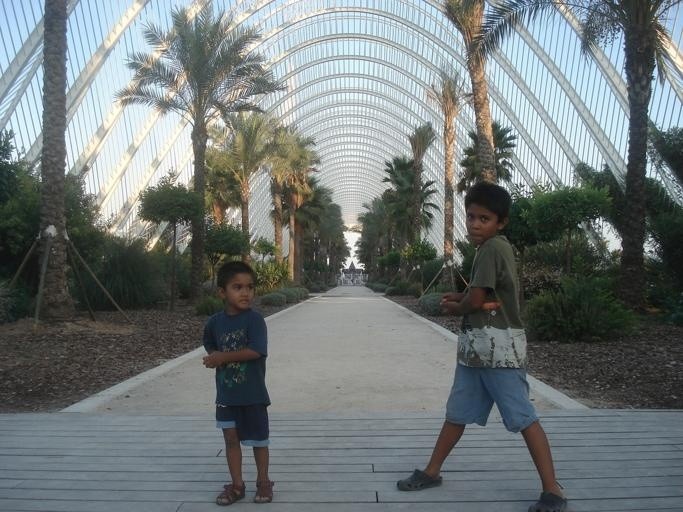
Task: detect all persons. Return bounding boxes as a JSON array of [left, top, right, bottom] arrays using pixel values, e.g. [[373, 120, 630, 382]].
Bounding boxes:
[[200, 259, 275, 506], [393, 180, 568, 511]]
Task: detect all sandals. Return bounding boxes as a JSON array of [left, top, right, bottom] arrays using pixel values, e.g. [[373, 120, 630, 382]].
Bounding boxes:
[[253, 478, 275, 503], [215, 480, 247, 505]]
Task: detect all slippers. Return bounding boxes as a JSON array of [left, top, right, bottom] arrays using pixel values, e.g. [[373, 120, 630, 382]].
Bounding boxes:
[[526, 490, 567, 512], [397, 467, 444, 491]]
